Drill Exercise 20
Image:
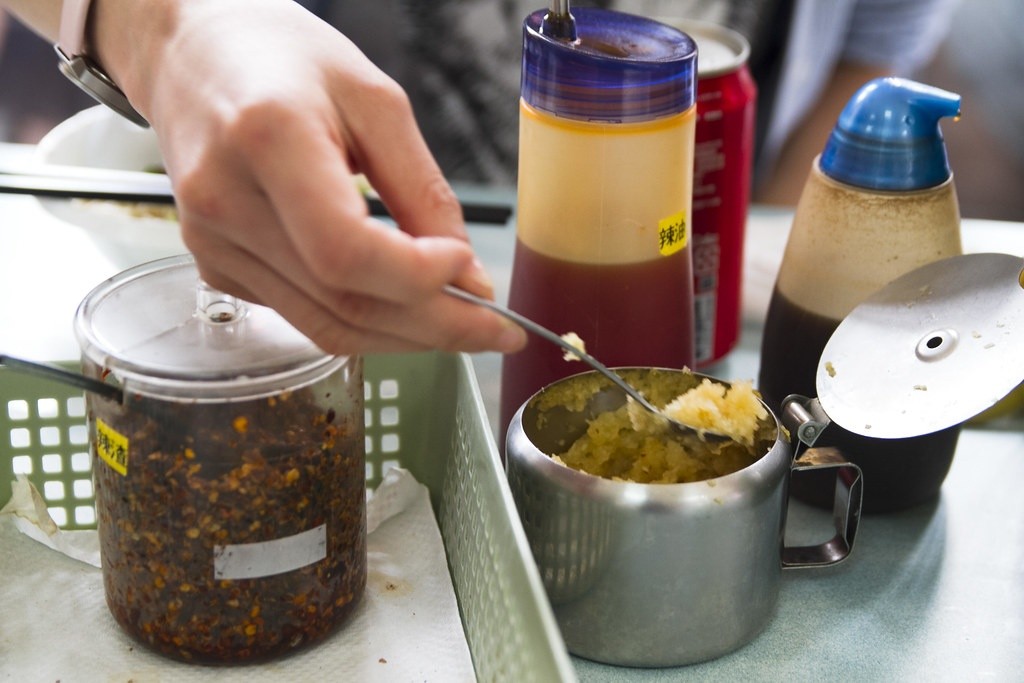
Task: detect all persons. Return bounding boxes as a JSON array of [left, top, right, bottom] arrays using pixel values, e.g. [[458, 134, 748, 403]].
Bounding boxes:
[[2, 0, 952, 358]]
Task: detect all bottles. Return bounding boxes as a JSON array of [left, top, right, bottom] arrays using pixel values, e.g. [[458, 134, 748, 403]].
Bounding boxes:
[[501, 1, 707, 454], [764, 76, 966, 510]]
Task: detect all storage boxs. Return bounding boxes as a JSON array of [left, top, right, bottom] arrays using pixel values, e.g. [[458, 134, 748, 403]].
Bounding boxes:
[[1, 350, 582, 683]]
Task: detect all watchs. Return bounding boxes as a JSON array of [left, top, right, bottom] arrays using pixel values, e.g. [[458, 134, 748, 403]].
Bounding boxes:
[[51, 1, 155, 136]]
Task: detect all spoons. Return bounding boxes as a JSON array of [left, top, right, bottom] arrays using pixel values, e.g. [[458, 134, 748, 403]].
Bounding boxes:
[[422, 284, 773, 447]]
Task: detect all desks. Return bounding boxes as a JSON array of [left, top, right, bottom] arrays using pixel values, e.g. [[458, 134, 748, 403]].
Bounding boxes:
[[1, 136, 1024, 683]]
